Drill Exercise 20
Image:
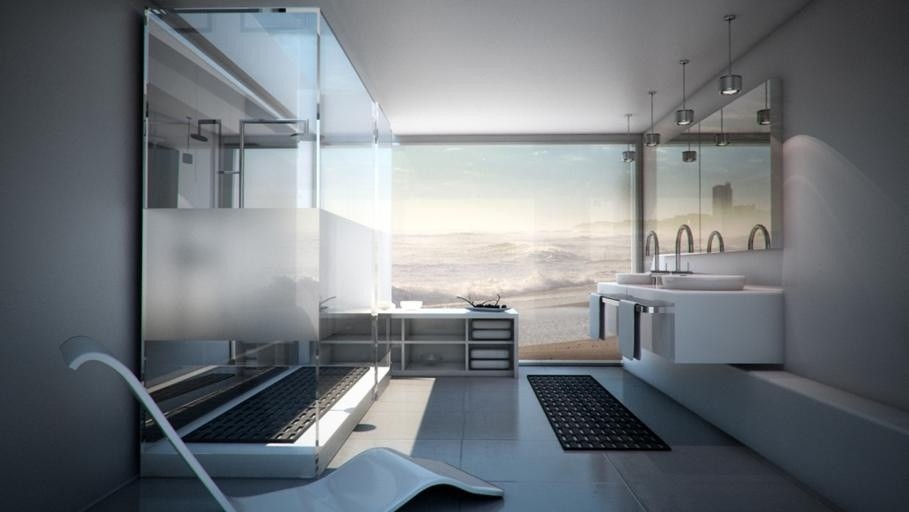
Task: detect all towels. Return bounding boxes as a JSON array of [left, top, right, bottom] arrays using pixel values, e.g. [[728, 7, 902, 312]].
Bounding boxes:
[[618, 299, 642, 361], [585, 292, 607, 339]]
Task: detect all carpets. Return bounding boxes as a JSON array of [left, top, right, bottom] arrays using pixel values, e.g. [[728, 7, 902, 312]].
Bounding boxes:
[[178, 361, 370, 449], [142, 362, 289, 444], [525, 372, 676, 453]]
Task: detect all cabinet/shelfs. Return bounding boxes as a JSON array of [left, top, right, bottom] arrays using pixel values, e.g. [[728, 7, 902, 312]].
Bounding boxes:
[[300, 305, 517, 381]]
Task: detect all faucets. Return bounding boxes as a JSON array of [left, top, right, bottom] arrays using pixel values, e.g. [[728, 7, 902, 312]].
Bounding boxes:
[[747, 224, 770, 250], [645, 231, 660, 271], [707, 230, 724, 252], [674, 224, 693, 272]]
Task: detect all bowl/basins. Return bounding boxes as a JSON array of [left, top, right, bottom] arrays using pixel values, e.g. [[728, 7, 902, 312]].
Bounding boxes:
[[400, 300, 423, 311]]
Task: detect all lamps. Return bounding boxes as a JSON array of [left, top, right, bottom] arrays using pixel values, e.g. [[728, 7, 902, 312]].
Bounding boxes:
[[621, 91, 660, 162], [681, 82, 770, 164], [675, 13, 744, 125]]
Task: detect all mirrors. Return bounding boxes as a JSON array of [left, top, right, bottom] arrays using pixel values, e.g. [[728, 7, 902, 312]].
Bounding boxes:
[[654, 77, 785, 256]]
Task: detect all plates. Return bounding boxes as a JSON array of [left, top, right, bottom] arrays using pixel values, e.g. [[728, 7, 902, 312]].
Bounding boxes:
[[466, 305, 511, 312]]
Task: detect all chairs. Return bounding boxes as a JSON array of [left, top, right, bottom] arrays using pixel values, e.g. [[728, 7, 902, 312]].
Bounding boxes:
[[58, 335, 505, 511]]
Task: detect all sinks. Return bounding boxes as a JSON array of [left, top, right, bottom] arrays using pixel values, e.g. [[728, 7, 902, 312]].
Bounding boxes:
[[661, 274, 746, 291], [617, 272, 651, 283]]
